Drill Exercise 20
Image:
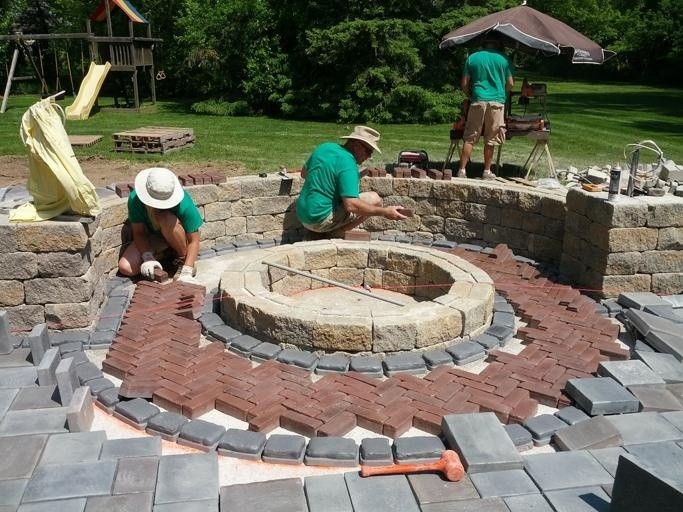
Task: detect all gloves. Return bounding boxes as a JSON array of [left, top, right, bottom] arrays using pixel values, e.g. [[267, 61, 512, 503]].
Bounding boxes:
[[140, 251, 163, 279], [176, 265, 198, 285]]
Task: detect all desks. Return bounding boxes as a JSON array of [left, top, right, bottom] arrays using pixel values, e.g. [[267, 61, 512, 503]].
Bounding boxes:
[[442, 129, 558, 185]]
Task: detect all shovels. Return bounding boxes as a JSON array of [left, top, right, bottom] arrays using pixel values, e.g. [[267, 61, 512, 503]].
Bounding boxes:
[[275, 168, 293, 180]]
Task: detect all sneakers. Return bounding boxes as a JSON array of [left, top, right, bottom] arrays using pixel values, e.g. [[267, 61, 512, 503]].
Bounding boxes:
[[302, 230, 320, 241], [173, 262, 197, 281], [457, 171, 495, 180]]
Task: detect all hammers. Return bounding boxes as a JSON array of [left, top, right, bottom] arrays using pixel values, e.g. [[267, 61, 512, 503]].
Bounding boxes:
[[361, 450, 465, 482]]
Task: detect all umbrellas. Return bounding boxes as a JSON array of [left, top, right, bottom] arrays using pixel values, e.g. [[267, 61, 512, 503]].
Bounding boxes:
[[439, 0, 617, 68]]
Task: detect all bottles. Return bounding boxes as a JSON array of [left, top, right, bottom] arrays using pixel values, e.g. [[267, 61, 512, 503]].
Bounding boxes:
[[608, 167, 621, 201]]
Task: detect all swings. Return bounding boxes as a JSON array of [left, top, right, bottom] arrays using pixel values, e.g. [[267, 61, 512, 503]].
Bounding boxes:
[[37, 40, 64, 100], [66, 39, 84, 100]]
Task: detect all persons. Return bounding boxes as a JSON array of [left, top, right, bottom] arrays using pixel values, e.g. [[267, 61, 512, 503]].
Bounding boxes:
[[119, 167, 202, 284], [458, 39, 513, 179], [295, 126, 407, 238]]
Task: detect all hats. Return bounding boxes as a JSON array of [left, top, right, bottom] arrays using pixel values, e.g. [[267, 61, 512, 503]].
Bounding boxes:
[[481, 31, 504, 42], [340, 125, 382, 154], [134, 167, 185, 210]]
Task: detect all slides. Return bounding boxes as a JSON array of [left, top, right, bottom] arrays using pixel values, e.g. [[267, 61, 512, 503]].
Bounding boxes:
[[65, 61, 112, 120]]
